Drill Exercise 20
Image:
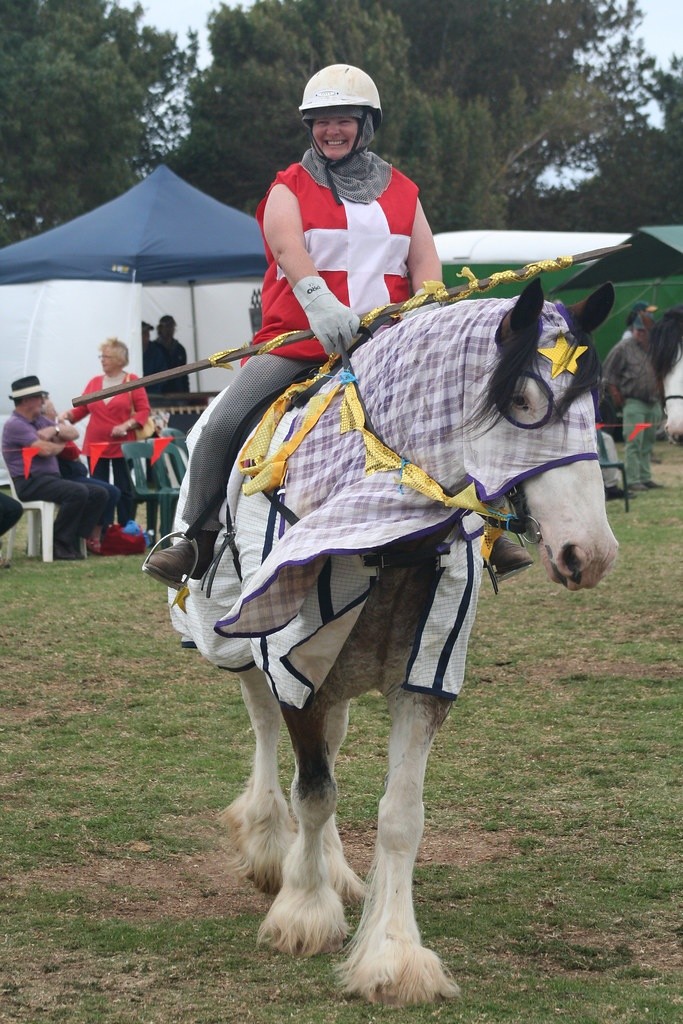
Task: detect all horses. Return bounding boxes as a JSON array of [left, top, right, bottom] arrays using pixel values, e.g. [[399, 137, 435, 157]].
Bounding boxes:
[[158, 273, 681, 1005]]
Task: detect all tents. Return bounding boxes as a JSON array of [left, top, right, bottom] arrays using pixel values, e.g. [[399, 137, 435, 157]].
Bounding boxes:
[[0, 164, 683, 487]]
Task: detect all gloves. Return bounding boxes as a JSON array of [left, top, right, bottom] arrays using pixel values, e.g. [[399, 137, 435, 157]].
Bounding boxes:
[[292, 276, 361, 355]]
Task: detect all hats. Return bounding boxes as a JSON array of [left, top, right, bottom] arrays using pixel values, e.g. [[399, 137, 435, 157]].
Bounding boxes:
[[633, 313, 656, 330], [8, 376, 49, 398], [632, 300, 657, 312]]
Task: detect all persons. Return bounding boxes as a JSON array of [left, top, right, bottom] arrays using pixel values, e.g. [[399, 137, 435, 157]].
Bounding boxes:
[[596, 428, 636, 502], [141, 319, 170, 395], [2, 375, 108, 560], [0, 492, 25, 538], [153, 314, 192, 395], [601, 310, 667, 492], [58, 336, 151, 528], [143, 62, 534, 582], [39, 395, 120, 557]]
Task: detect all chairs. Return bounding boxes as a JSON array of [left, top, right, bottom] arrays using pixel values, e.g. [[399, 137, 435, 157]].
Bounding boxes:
[[1, 450, 87, 564], [597, 429, 629, 512], [120, 428, 189, 549]]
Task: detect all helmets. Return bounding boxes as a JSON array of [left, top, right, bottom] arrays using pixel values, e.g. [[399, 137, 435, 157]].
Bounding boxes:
[[298, 63, 382, 111]]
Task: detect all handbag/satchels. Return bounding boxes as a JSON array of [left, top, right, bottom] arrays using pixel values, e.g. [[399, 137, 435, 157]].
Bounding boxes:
[[101, 520, 146, 554], [142, 528, 153, 547], [131, 411, 155, 439]]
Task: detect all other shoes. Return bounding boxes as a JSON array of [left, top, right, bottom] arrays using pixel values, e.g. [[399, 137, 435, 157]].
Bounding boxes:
[[645, 480, 662, 487], [53, 541, 75, 559], [0, 559, 12, 568], [606, 486, 636, 499], [488, 534, 533, 582], [74, 550, 85, 559], [628, 484, 649, 490], [144, 538, 214, 583]]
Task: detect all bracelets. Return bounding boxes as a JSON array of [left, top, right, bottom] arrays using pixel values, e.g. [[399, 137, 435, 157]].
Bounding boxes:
[[126, 421, 132, 430]]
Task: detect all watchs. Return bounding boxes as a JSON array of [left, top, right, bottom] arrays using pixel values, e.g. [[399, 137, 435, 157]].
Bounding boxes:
[[53, 424, 59, 437]]
